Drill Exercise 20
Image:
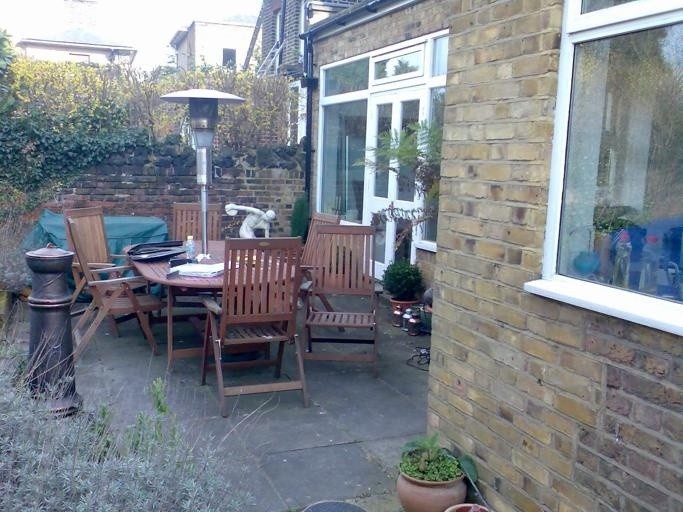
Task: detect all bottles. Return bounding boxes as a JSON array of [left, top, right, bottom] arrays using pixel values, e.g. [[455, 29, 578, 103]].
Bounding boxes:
[[612, 232, 658, 295], [185, 235, 195, 262], [392, 305, 421, 335]]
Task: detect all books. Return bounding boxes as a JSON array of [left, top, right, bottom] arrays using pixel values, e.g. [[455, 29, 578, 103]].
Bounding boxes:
[[170, 262, 224, 278]]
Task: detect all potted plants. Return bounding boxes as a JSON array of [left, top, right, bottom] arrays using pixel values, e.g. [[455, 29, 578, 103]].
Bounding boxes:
[[379, 258, 427, 326], [395, 432, 468, 512]]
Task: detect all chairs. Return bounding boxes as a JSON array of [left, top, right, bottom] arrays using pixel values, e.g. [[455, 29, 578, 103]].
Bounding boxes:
[[299, 223, 379, 371], [287, 212, 346, 333], [155, 204, 224, 331], [63, 207, 162, 360], [201, 235, 310, 418]]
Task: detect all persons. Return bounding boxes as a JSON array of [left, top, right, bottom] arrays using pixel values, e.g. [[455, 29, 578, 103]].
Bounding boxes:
[[223, 200, 276, 239]]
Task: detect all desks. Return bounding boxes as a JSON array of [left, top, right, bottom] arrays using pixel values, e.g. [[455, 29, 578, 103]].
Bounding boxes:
[[113, 237, 297, 378]]
